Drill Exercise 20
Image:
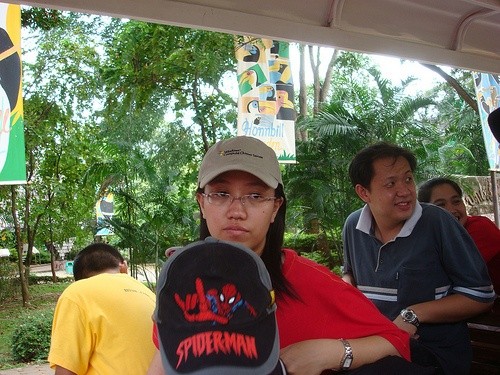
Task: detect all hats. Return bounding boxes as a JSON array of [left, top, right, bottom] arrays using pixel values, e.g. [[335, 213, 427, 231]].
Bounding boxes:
[[152, 237, 280, 374], [198, 136, 283, 189]]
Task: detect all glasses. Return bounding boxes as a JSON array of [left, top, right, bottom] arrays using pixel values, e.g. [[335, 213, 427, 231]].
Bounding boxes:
[[202, 193, 278, 207]]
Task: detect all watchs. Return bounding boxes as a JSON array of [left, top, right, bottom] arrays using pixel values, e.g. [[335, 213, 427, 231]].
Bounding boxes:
[[400, 308, 419, 328], [331, 338, 353, 372]]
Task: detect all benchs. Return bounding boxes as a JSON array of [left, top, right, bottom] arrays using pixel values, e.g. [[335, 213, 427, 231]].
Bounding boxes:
[[466, 318, 500, 375]]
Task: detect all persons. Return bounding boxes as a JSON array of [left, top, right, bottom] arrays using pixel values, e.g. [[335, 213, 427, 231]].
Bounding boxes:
[[418, 178, 500, 315], [340, 142, 496, 375], [48, 243, 157, 375], [149, 135, 411, 375]]
